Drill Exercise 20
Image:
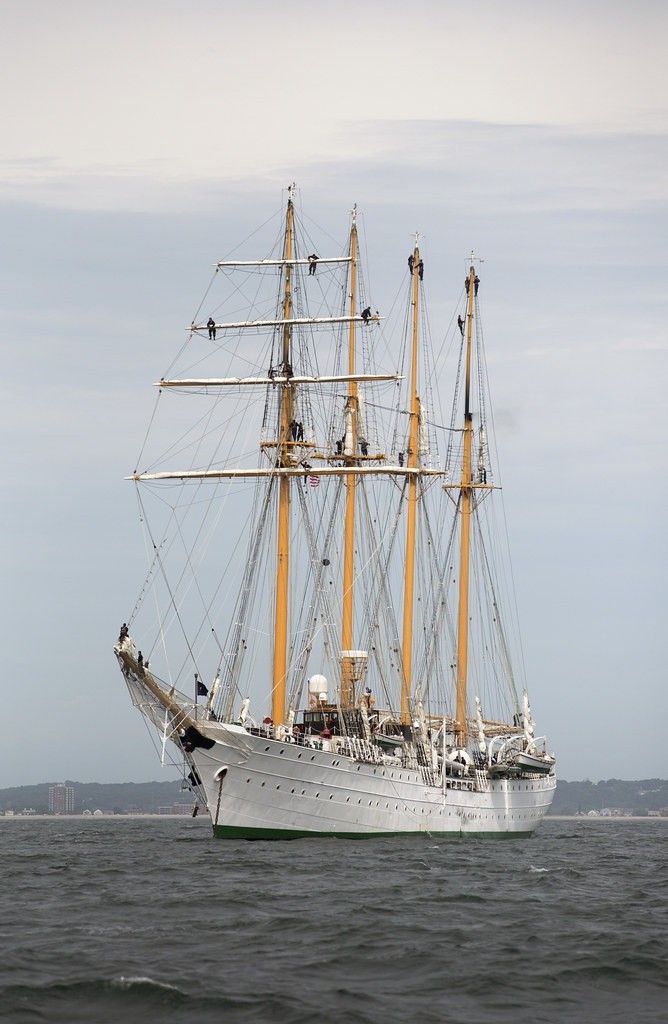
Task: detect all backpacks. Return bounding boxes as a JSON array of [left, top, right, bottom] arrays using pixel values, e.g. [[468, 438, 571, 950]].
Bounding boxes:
[[121, 627, 127, 636]]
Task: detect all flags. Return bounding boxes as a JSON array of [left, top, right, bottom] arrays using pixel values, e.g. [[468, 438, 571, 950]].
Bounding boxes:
[[196, 681, 208, 696]]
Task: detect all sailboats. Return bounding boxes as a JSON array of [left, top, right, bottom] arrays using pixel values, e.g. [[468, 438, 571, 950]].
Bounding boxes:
[[104, 181, 561, 842]]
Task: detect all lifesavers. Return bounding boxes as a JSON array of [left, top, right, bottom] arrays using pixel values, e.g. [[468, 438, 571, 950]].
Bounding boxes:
[[294, 727, 301, 733]]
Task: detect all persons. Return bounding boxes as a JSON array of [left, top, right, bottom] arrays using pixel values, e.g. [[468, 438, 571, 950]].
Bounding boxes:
[[119, 623, 129, 644], [398, 452, 404, 467], [513, 713, 519, 727], [300, 462, 312, 484], [208, 710, 217, 721], [207, 317, 216, 340], [289, 419, 303, 442], [268, 367, 278, 389], [361, 307, 371, 326], [458, 315, 465, 336], [473, 275, 480, 297], [137, 651, 143, 666], [464, 276, 470, 298], [188, 766, 202, 797], [366, 687, 372, 709], [279, 360, 294, 385], [407, 254, 414, 275], [414, 259, 423, 281], [307, 254, 319, 276], [453, 756, 466, 779], [335, 433, 346, 456], [359, 440, 370, 456], [482, 468, 487, 484]]
